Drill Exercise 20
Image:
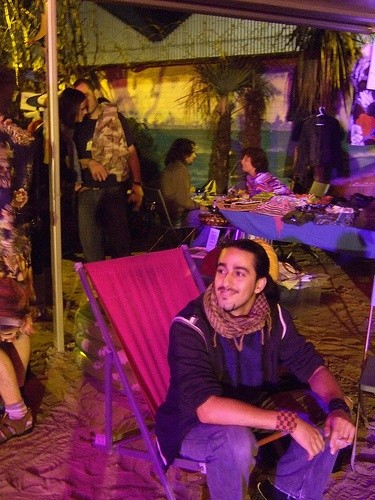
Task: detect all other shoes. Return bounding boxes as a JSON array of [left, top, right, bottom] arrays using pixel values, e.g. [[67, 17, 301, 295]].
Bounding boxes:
[[62, 298, 75, 308], [36, 308, 53, 320]]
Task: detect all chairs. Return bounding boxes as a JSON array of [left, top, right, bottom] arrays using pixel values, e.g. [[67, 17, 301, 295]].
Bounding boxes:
[[350, 278, 375, 477], [138, 182, 200, 251], [277, 180, 332, 262], [78, 245, 291, 500]]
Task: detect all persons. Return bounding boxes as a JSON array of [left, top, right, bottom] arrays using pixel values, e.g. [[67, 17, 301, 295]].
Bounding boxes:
[[31, 87, 88, 321], [154, 238, 356, 500], [0, 113, 35, 440], [228, 147, 295, 195], [74, 79, 144, 261], [155, 138, 219, 246]]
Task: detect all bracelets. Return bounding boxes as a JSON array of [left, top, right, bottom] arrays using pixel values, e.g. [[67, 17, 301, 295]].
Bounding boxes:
[[132, 181, 141, 185], [329, 398, 350, 413], [275, 410, 297, 433]]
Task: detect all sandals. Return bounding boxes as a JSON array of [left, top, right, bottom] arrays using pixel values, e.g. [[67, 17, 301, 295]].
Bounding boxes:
[[0, 408, 33, 444]]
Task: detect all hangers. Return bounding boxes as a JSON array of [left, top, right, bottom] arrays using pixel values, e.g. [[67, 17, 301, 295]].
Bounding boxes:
[[316, 105, 327, 127]]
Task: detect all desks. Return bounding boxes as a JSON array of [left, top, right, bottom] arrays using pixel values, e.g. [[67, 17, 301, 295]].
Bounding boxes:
[[219, 208, 375, 285]]
